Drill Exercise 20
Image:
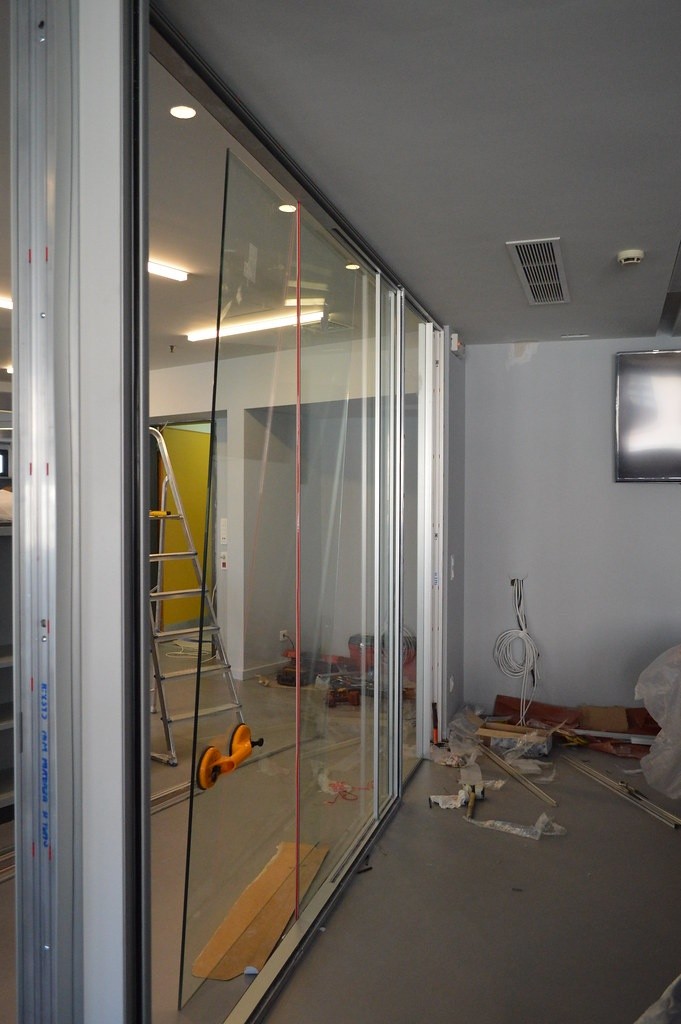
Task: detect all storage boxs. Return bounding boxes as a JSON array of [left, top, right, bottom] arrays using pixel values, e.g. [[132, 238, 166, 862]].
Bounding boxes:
[[280, 630, 288, 641], [467, 711, 567, 756]]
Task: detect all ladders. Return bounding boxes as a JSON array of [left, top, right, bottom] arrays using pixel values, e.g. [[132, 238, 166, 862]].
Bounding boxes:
[[145, 424, 246, 768]]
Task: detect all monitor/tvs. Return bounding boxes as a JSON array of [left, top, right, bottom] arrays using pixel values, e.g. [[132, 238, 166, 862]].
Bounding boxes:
[[614, 349, 681, 483]]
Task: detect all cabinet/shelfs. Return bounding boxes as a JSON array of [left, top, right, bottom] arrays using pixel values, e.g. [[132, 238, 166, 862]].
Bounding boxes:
[[0, 530, 15, 884]]
